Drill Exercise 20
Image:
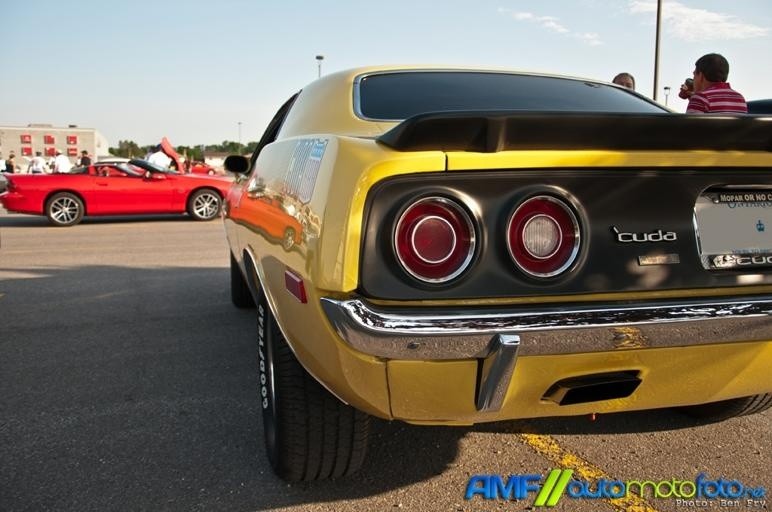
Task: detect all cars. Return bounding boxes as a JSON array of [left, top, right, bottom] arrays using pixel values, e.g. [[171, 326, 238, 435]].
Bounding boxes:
[[0, 137, 230, 227], [221, 63, 772, 486]]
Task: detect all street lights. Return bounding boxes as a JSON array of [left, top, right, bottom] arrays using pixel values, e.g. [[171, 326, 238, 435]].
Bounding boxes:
[[315, 54, 324, 76]]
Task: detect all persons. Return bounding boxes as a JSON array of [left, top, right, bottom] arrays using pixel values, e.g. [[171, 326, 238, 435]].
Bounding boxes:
[[612, 71, 636, 91], [26, 150, 49, 174], [148, 143, 169, 169], [5, 154, 17, 173], [74, 149, 91, 166], [679, 52, 747, 115], [183, 157, 192, 173], [53, 149, 71, 172], [143, 146, 154, 160], [45, 162, 49, 170]]
